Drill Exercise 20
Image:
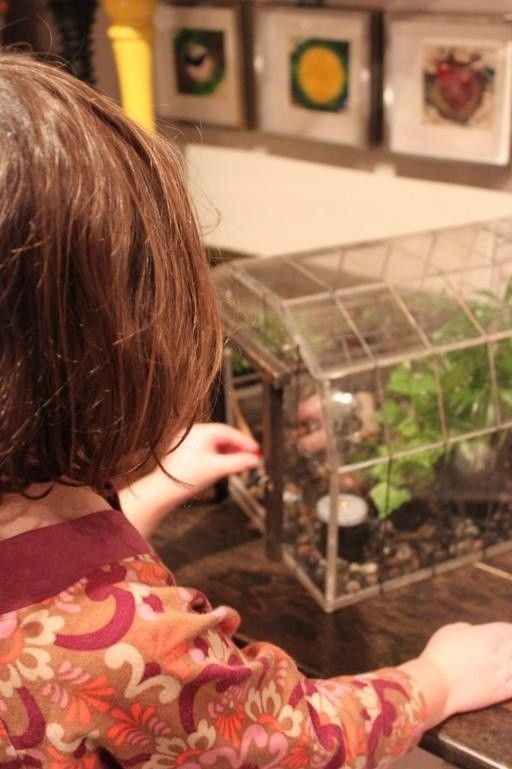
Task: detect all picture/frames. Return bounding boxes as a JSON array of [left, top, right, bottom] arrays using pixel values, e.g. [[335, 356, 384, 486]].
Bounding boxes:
[[146, 1, 511, 170]]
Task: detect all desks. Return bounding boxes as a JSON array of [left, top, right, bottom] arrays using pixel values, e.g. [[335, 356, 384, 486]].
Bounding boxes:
[[114, 244, 508, 769]]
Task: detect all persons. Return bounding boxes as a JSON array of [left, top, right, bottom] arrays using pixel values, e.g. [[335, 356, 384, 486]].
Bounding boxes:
[[0, 56, 512, 769]]
